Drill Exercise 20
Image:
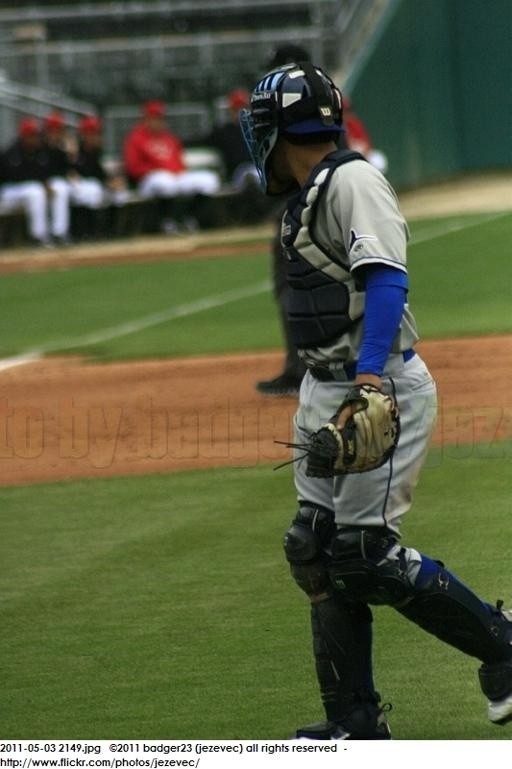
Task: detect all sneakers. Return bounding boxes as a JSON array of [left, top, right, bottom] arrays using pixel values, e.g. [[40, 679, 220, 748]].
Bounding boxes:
[[256, 365, 300, 392], [488, 610, 512, 726], [295, 722, 392, 740]]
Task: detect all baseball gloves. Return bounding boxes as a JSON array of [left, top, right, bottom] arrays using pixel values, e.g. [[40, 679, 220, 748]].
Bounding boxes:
[[273, 384, 400, 478]]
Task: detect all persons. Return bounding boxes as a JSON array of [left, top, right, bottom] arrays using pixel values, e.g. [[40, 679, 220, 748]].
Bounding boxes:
[[237, 62, 511, 740], [251, 45, 373, 398]]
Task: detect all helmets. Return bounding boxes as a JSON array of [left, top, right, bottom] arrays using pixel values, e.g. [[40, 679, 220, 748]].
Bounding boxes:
[[248, 63, 349, 141]]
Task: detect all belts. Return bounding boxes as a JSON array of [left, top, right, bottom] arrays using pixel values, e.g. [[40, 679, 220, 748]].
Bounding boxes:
[[301, 360, 359, 384]]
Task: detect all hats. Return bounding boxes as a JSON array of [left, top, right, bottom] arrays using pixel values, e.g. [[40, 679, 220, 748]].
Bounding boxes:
[[341, 96, 353, 113], [233, 90, 249, 111], [18, 111, 101, 133], [143, 101, 165, 118]]
